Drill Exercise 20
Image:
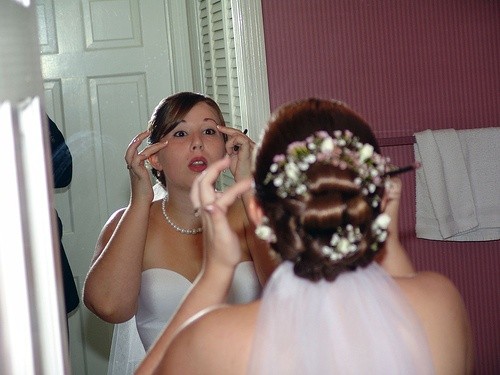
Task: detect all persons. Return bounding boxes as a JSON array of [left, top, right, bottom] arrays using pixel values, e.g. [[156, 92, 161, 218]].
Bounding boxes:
[[131, 98, 477, 374], [83, 92, 280, 374], [45, 111, 80, 349]]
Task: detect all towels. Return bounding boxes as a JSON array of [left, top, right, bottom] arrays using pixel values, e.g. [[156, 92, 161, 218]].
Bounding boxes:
[[413, 127, 500, 242]]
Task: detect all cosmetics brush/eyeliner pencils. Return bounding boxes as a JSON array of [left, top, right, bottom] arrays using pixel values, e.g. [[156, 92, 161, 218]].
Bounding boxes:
[[382, 161, 420, 178], [234, 128, 247, 152]]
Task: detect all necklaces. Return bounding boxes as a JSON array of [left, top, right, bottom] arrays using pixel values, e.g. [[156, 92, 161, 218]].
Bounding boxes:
[[161, 196, 202, 235]]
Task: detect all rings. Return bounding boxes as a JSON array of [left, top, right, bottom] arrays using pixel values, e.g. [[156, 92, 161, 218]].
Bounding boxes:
[[127, 165, 132, 170], [194, 208, 200, 218]]
